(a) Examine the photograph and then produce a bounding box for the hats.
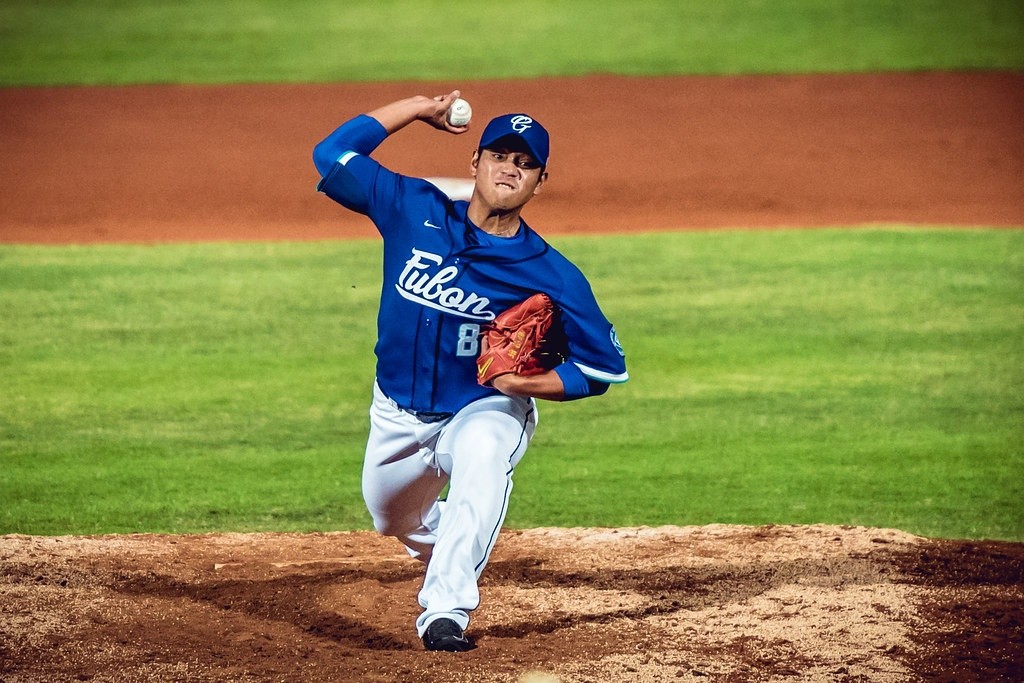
[479,113,550,168]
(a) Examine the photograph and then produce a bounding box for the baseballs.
[445,98,473,127]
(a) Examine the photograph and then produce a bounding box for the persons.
[315,90,629,653]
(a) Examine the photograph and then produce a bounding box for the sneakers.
[423,618,472,651]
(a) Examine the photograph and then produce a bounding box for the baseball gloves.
[473,290,556,390]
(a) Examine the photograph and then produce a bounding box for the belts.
[398,408,452,424]
